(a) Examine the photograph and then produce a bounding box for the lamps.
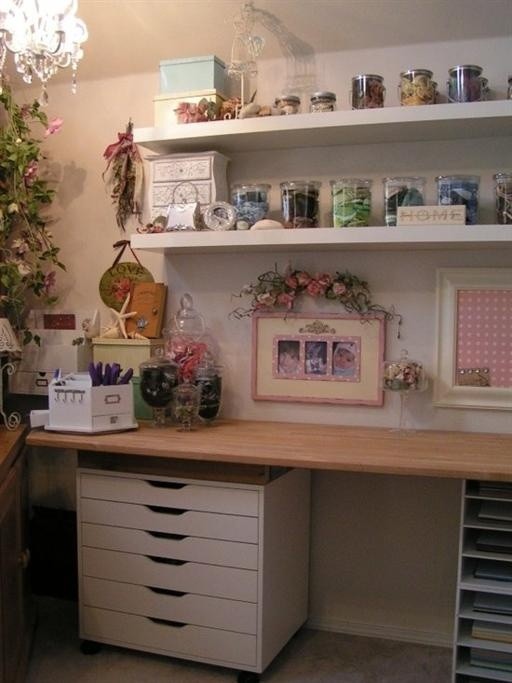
[1,315,24,430]
[1,0,91,105]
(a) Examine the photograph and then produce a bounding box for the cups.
[229,172,512,232]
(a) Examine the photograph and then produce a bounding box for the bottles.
[159,292,219,425]
[274,64,491,113]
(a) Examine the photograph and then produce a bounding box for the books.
[465,478,512,673]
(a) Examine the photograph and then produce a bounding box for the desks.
[26,410,512,682]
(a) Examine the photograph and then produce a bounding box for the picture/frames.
[250,310,389,407]
[430,266,512,409]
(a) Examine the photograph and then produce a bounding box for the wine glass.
[139,347,178,430]
[188,350,223,427]
[174,377,199,433]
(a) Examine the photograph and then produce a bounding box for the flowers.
[172,96,221,123]
[227,256,405,325]
[379,356,429,393]
[0,90,67,365]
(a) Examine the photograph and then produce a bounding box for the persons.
[333,341,358,378]
[305,341,327,374]
[278,340,298,373]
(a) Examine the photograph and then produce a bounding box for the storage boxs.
[151,87,229,124]
[158,54,231,96]
[131,377,155,420]
[90,336,166,375]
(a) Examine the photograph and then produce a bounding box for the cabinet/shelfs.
[145,148,230,229]
[0,426,33,682]
[450,478,512,683]
[130,96,512,251]
[75,465,315,681]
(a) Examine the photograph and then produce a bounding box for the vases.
[396,390,417,437]
